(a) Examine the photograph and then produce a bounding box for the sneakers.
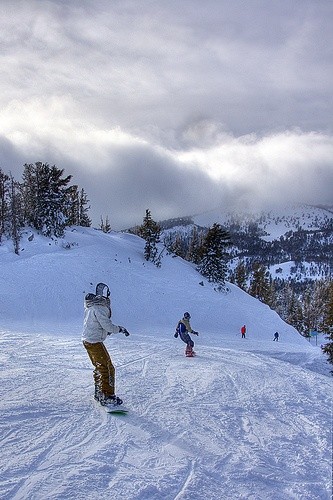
[186,346,193,357]
[95,385,103,402]
[100,395,123,407]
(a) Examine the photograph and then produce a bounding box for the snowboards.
[92,395,129,413]
[185,352,195,357]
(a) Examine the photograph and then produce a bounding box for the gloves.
[118,326,129,336]
[174,333,178,338]
[193,331,198,336]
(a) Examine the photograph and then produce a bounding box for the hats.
[184,312,190,317]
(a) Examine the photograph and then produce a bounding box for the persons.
[240,324,247,338]
[272,331,279,342]
[174,311,198,357]
[80,282,130,408]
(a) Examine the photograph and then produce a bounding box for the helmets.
[96,283,110,298]
